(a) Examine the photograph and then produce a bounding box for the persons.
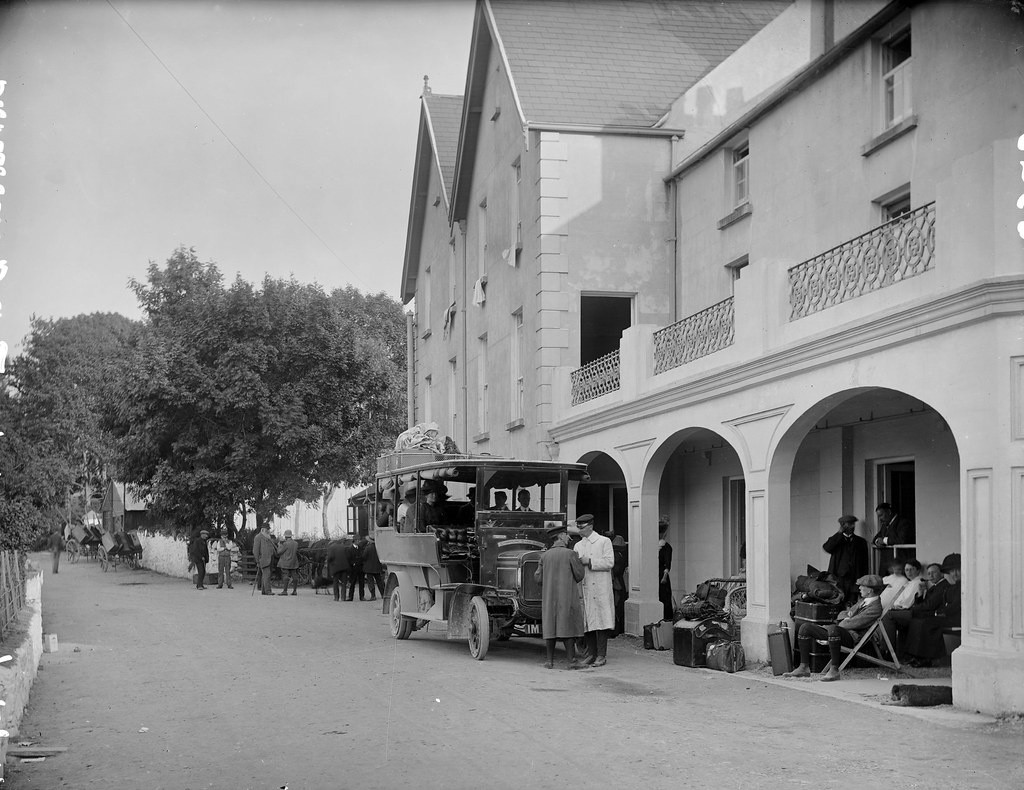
[48,527,63,573]
[659,521,674,620]
[277,530,299,596]
[253,524,276,595]
[192,530,209,590]
[872,503,911,589]
[514,490,533,511]
[534,525,589,669]
[573,514,614,666]
[783,553,961,682]
[602,530,628,640]
[327,535,385,601]
[216,530,233,589]
[740,541,746,566]
[486,491,510,511]
[377,486,449,532]
[822,515,868,604]
[459,487,476,527]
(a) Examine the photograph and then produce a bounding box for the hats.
[421,481,444,494]
[366,533,375,543]
[856,575,883,587]
[260,524,293,536]
[547,514,593,539]
[405,490,416,497]
[466,488,475,497]
[839,515,857,523]
[940,554,961,571]
[200,530,227,534]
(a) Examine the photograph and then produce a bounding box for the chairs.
[815,583,916,678]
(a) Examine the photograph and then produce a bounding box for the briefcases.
[643,619,675,651]
[767,621,793,676]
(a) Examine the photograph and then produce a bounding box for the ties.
[846,537,850,543]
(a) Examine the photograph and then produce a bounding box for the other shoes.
[580,655,596,667]
[198,585,297,596]
[905,657,937,668]
[821,670,840,681]
[564,658,589,670]
[783,664,810,677]
[334,597,383,601]
[592,656,607,667]
[544,662,552,668]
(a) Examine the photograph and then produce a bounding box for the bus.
[369,459,581,662]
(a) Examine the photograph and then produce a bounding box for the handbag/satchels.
[696,580,727,608]
[706,639,745,672]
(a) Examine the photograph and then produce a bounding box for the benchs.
[426,524,479,562]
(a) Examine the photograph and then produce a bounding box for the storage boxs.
[671,617,732,669]
[378,449,433,473]
